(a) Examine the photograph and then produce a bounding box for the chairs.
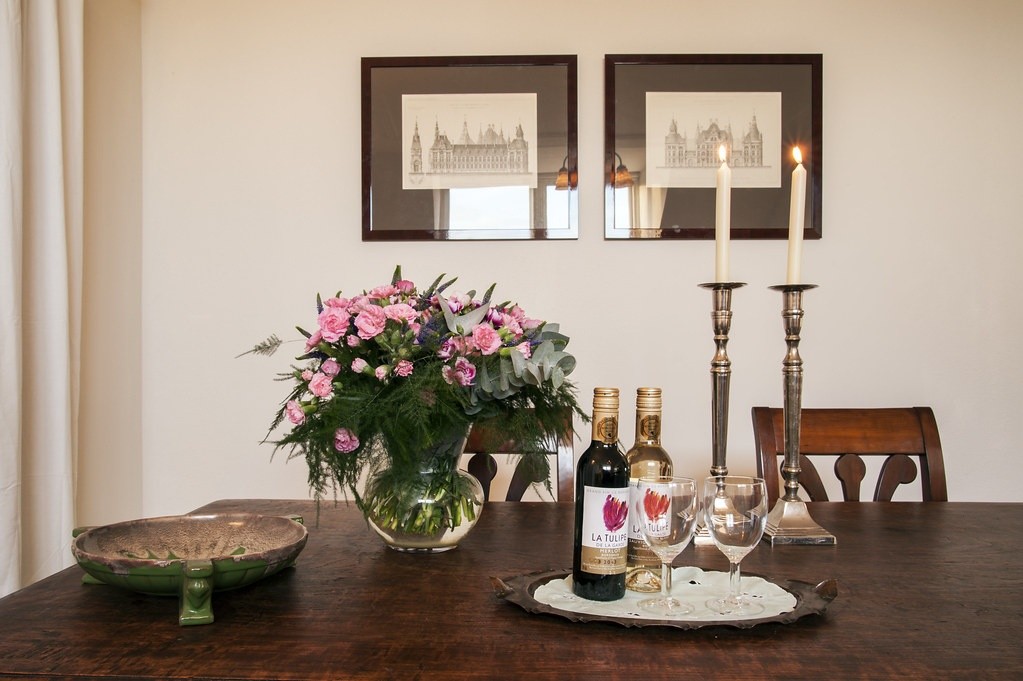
[752,407,948,502]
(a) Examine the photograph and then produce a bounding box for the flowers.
[235,265,593,535]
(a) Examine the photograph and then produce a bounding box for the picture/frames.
[605,54,822,240]
[361,55,578,242]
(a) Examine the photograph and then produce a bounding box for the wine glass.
[636,477,700,617]
[704,476,768,615]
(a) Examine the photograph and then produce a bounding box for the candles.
[786,145,807,284]
[716,145,731,282]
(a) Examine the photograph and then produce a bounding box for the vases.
[362,423,485,552]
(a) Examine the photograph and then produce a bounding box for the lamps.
[611,151,635,187]
[555,156,577,190]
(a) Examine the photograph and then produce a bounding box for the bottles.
[622,387,673,592]
[571,388,630,602]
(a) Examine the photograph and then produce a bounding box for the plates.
[71,513,310,626]
[489,567,838,630]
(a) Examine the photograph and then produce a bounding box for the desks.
[0,499,1023,681]
